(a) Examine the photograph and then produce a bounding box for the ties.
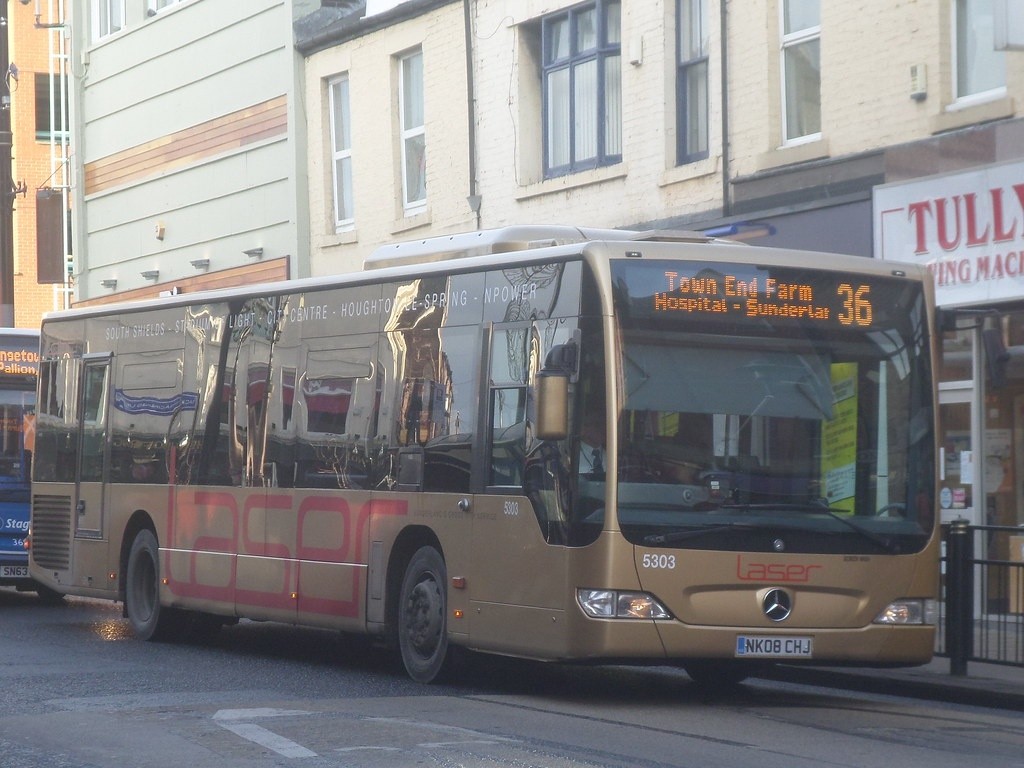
[592,449,604,474]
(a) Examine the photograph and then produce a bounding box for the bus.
[0,327,69,599]
[29,236,1012,695]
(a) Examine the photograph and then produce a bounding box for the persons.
[578,409,607,474]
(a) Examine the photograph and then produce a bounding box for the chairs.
[752,467,806,502]
[715,456,759,475]
[3,449,32,476]
